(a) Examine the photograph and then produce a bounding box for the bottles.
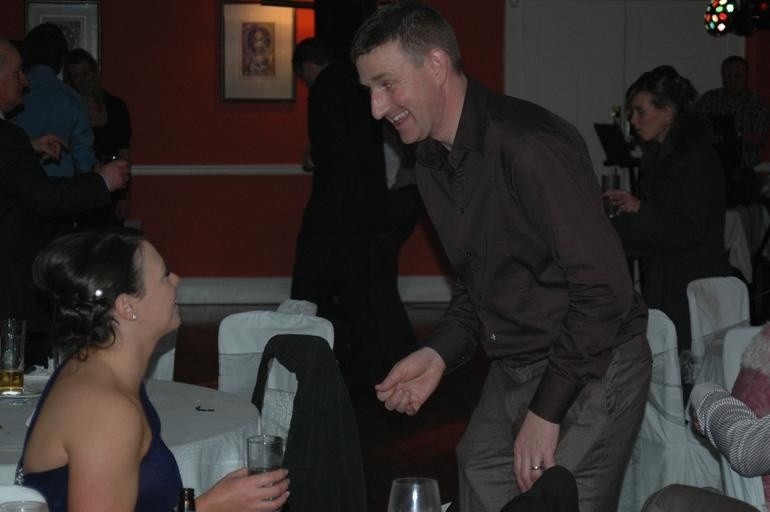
[176,488,196,512]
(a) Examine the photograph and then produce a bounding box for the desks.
[0,374,264,511]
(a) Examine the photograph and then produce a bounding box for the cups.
[602,174,621,220]
[388,478,441,512]
[0,318,27,395]
[0,500,48,512]
[246,435,284,512]
[106,150,128,164]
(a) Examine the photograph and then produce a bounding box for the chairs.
[250,333,370,512]
[718,326,766,508]
[217,311,337,402]
[617,306,724,511]
[140,302,179,379]
[686,274,751,386]
[0,485,49,511]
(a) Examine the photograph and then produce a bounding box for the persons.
[293,38,424,368]
[603,57,769,512]
[13,227,292,512]
[2,23,131,374]
[346,0,653,510]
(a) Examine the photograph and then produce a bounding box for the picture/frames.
[24,0,102,78]
[218,1,298,104]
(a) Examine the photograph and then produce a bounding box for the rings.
[531,465,541,469]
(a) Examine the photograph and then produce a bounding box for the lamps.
[703,0,742,38]
